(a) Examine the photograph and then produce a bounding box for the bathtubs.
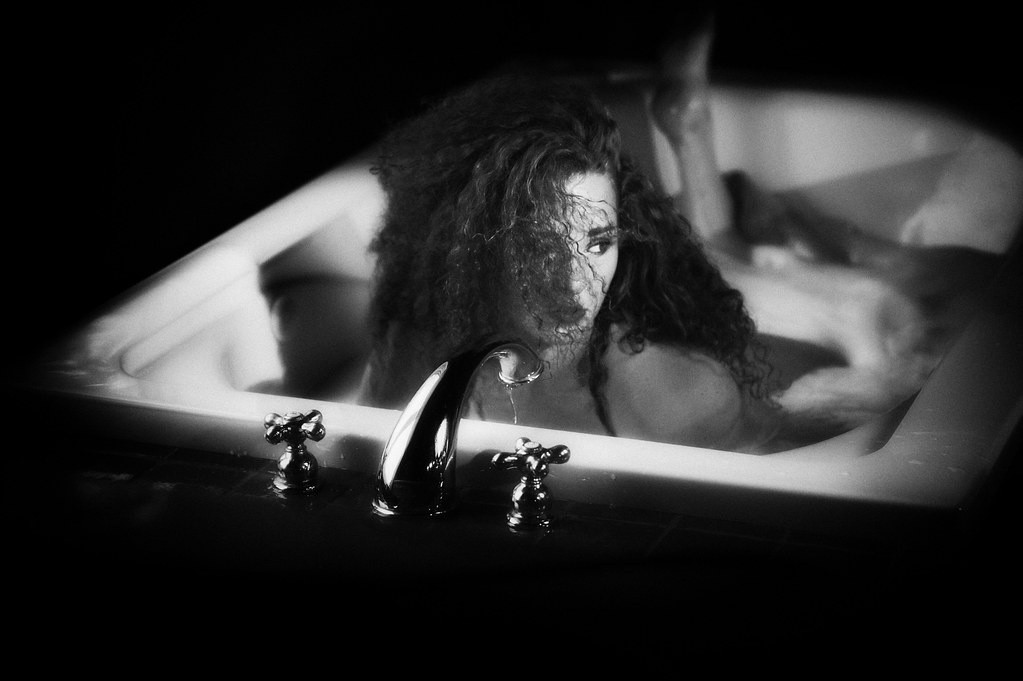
[28,76,1021,518]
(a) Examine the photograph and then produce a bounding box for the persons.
[362,19,769,447]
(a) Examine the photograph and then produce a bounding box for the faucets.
[366,330,547,523]
